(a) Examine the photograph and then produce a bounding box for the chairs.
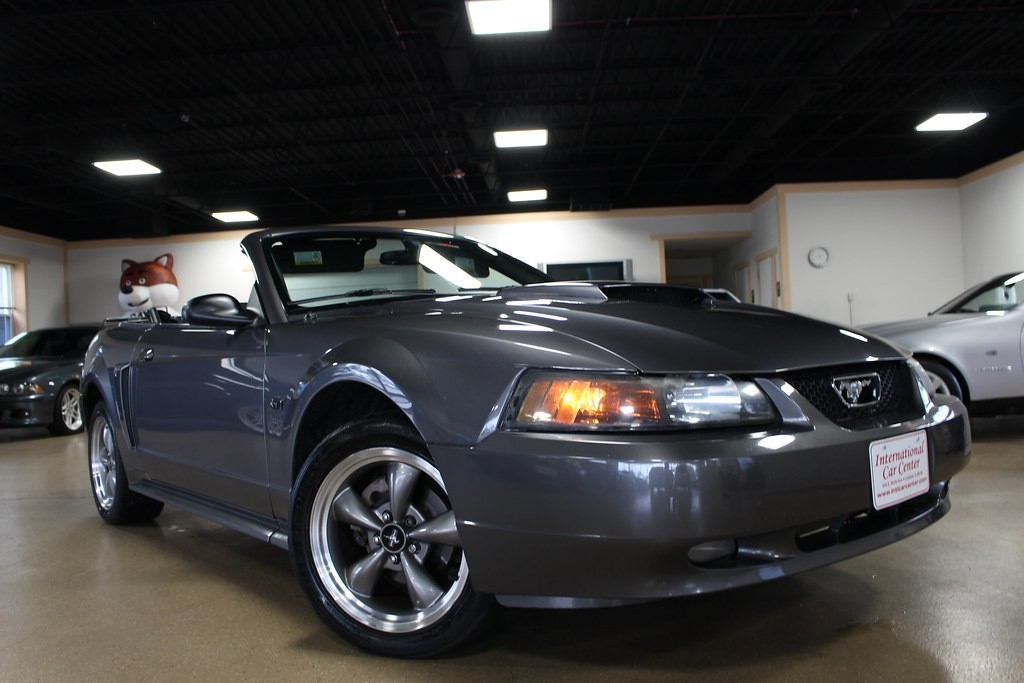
[181,293,245,327]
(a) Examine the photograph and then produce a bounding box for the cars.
[852,268,1024,417]
[0,318,114,434]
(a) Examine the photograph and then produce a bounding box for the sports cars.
[77,219,972,661]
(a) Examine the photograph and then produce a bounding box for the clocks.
[808,246,831,268]
